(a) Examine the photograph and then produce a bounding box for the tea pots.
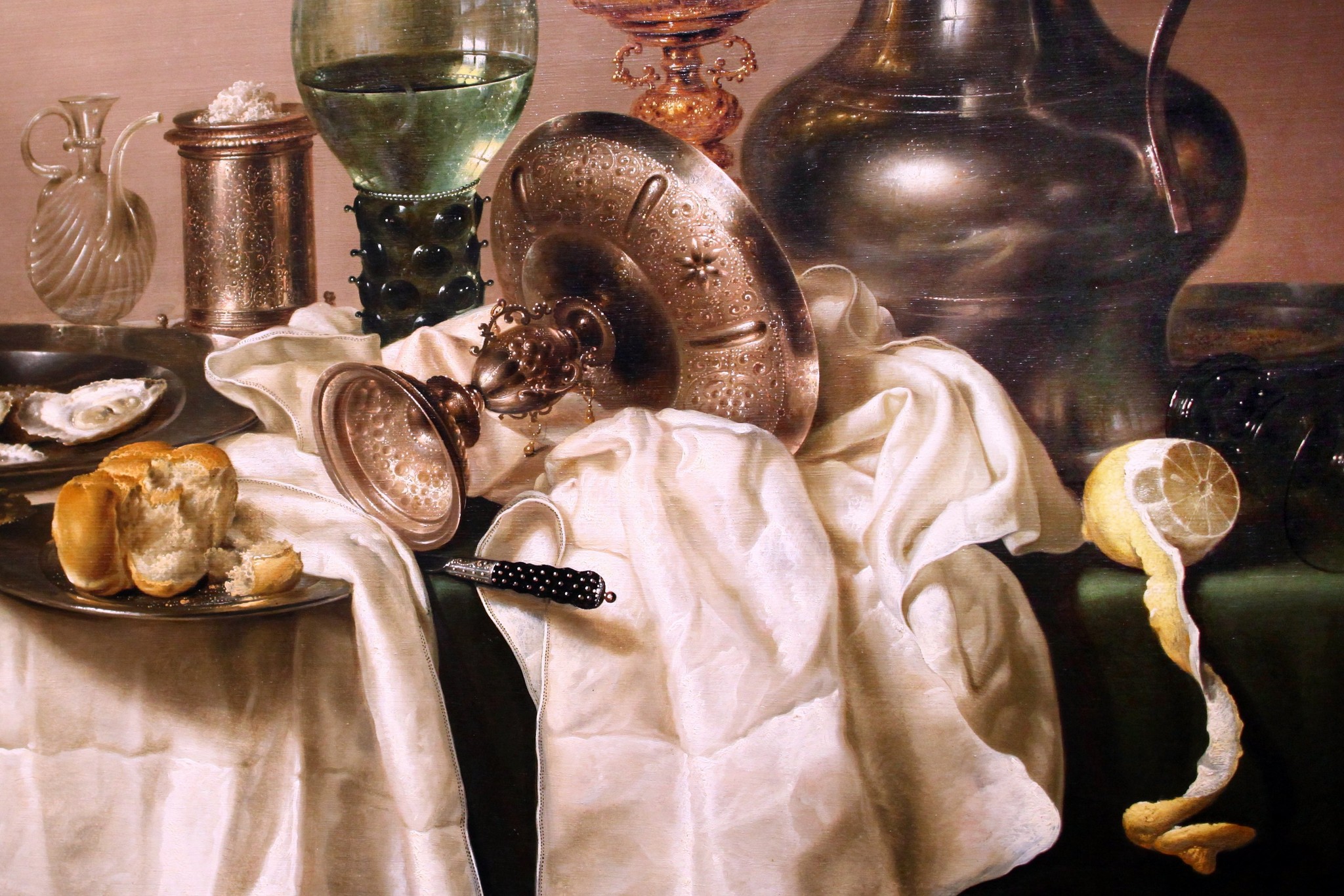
[20,93,161,327]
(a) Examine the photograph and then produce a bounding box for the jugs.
[737,0,1247,496]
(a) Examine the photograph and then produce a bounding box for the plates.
[1168,279,1344,365]
[0,502,353,622]
[0,321,257,476]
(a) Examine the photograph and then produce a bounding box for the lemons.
[1081,437,1239,569]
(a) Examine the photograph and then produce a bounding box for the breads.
[53,440,305,598]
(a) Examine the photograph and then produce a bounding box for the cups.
[165,102,323,337]
